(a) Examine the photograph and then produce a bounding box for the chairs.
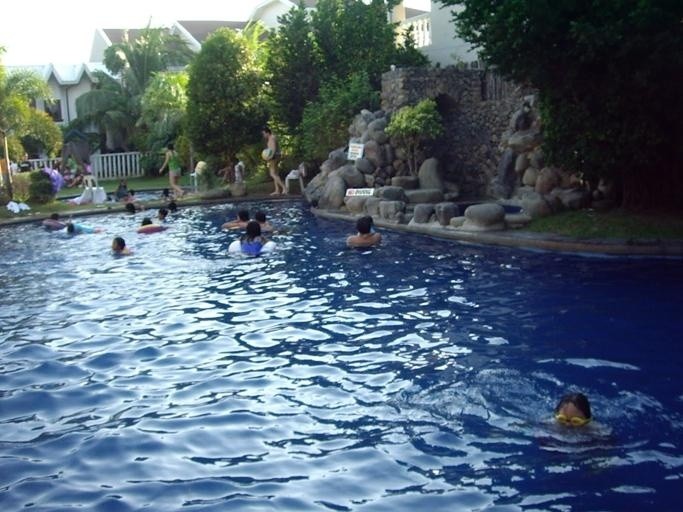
[82,176,105,205]
[284,162,307,194]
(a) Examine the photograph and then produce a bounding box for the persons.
[238,210,274,232]
[346,216,382,249]
[510,100,531,134]
[261,127,286,197]
[48,213,101,234]
[552,392,594,428]
[115,179,179,227]
[239,221,268,256]
[18,152,32,172]
[217,154,247,185]
[223,209,254,230]
[52,154,92,189]
[109,237,130,256]
[158,145,187,199]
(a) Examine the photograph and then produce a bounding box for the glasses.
[555,414,590,426]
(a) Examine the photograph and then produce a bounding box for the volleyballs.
[262,149,273,161]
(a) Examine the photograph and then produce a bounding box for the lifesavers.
[136,224,166,233]
[42,218,64,230]
[227,240,276,256]
